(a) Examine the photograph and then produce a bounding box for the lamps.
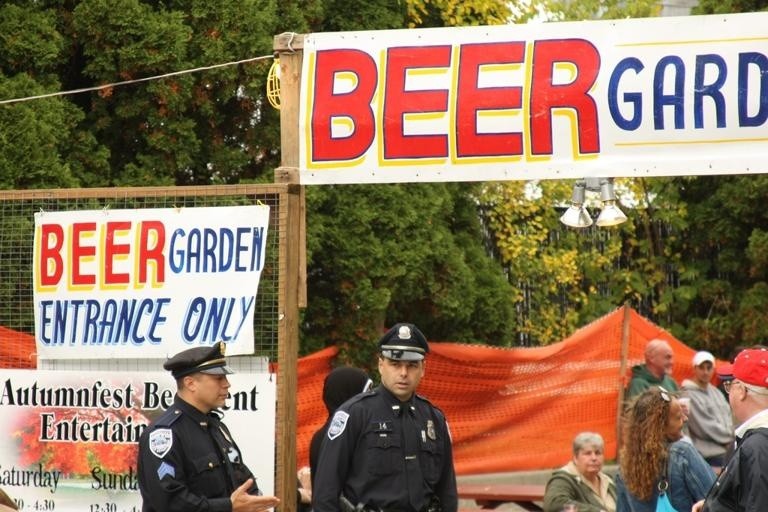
[558,177,627,229]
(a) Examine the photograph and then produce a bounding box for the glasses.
[658,385,671,412]
[723,380,743,394]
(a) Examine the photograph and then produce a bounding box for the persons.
[543,338,768,512]
[309,366,374,485]
[296,466,315,505]
[136,342,282,512]
[310,322,459,512]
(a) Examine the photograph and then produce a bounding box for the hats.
[716,347,768,389]
[379,322,429,361]
[693,350,715,367]
[163,341,235,379]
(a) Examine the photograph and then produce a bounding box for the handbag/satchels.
[654,487,678,512]
